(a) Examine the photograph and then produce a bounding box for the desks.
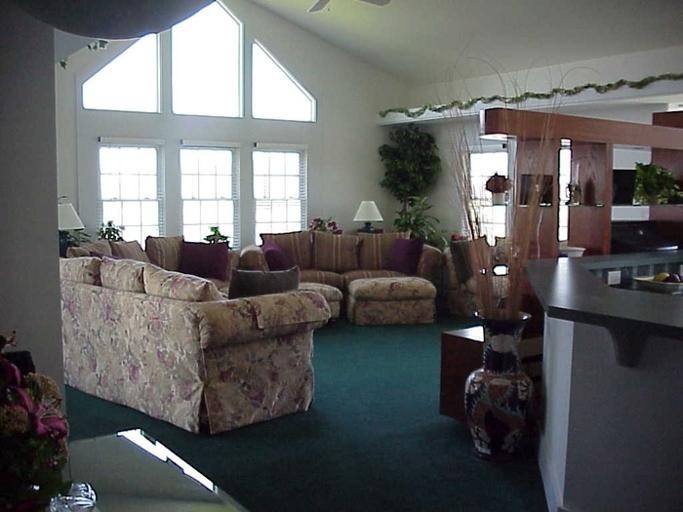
[357,229,383,233]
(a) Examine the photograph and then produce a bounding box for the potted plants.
[484,171,512,206]
[377,121,442,238]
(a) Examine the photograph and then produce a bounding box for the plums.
[665,272,681,282]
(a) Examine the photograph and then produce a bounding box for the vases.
[0,438,66,511]
[464,310,533,463]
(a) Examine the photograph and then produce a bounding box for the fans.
[307,0,392,13]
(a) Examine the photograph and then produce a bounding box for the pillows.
[263,241,293,270]
[390,240,418,274]
[229,265,300,307]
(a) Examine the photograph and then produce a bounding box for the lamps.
[351,198,385,231]
[58,203,85,257]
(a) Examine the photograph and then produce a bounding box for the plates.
[633,274,682,291]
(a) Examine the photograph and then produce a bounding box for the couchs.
[445,235,511,319]
[66,236,240,294]
[60,257,330,436]
[240,231,442,319]
[347,278,438,325]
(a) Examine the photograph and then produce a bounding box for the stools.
[298,280,343,323]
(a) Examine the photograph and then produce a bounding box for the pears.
[655,272,670,281]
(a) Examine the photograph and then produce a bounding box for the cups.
[490,191,511,206]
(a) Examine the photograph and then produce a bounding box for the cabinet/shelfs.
[439,324,540,440]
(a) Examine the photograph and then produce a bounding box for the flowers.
[0,331,73,462]
[311,216,343,236]
